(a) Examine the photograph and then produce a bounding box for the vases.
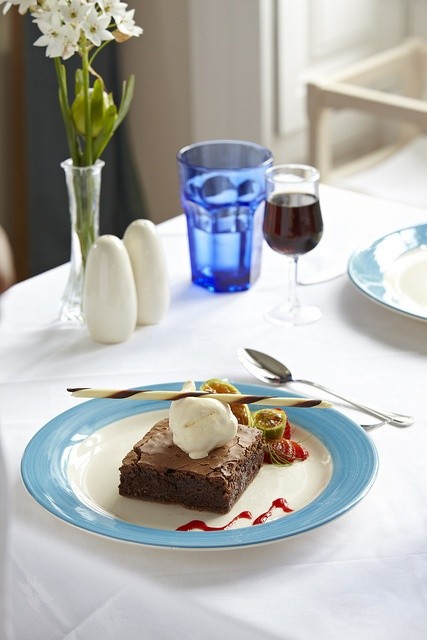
[56,156,106,325]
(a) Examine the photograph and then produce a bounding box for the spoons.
[238,346,416,427]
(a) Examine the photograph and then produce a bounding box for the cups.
[174,138,273,294]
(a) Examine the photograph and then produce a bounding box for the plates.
[345,219,427,322]
[19,376,381,552]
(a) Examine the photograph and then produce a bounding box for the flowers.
[0,0,143,165]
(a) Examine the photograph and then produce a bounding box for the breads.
[67,387,334,514]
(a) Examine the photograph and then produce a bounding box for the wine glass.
[262,163,324,329]
